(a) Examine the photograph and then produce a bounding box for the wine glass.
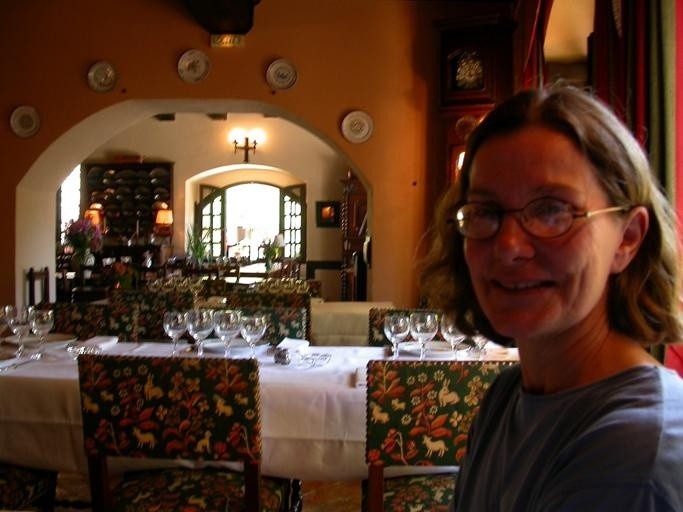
[160,307,267,358]
[382,312,488,362]
[3,304,53,361]
[144,276,203,300]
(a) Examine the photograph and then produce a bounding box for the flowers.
[64,218,102,254]
[260,233,284,276]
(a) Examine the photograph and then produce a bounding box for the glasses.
[450,195,626,240]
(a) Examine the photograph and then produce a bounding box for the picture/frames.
[314,200,341,229]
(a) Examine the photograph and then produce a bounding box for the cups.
[253,276,310,294]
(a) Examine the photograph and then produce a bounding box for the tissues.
[274,336,312,366]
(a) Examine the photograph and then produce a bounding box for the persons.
[409,82,682,511]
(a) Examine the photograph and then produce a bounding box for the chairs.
[365,305,521,511]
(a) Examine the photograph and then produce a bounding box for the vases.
[68,245,95,279]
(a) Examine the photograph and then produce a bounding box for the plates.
[198,335,270,354]
[396,339,470,357]
[5,333,77,351]
[88,165,170,233]
